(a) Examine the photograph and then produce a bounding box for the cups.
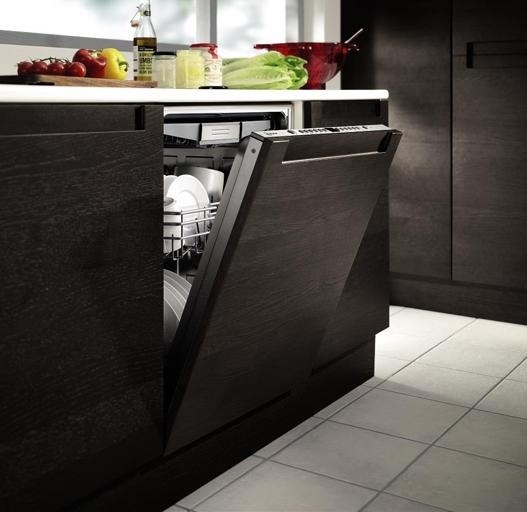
[151,50,177,88]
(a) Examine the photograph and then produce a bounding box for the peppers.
[101,47,129,80]
[72,48,107,78]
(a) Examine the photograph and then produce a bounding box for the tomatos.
[17,60,87,77]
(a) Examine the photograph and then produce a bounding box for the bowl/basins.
[254,42,359,90]
[174,166,225,202]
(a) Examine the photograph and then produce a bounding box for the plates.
[157,161,211,348]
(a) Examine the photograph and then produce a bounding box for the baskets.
[163,202,221,260]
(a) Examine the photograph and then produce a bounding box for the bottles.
[131,0,158,83]
[188,42,222,88]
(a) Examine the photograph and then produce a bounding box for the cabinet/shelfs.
[1,102,391,511]
[341,0,525,327]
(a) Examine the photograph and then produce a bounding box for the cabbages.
[222,51,308,90]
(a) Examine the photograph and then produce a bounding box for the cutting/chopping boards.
[0,73,159,88]
